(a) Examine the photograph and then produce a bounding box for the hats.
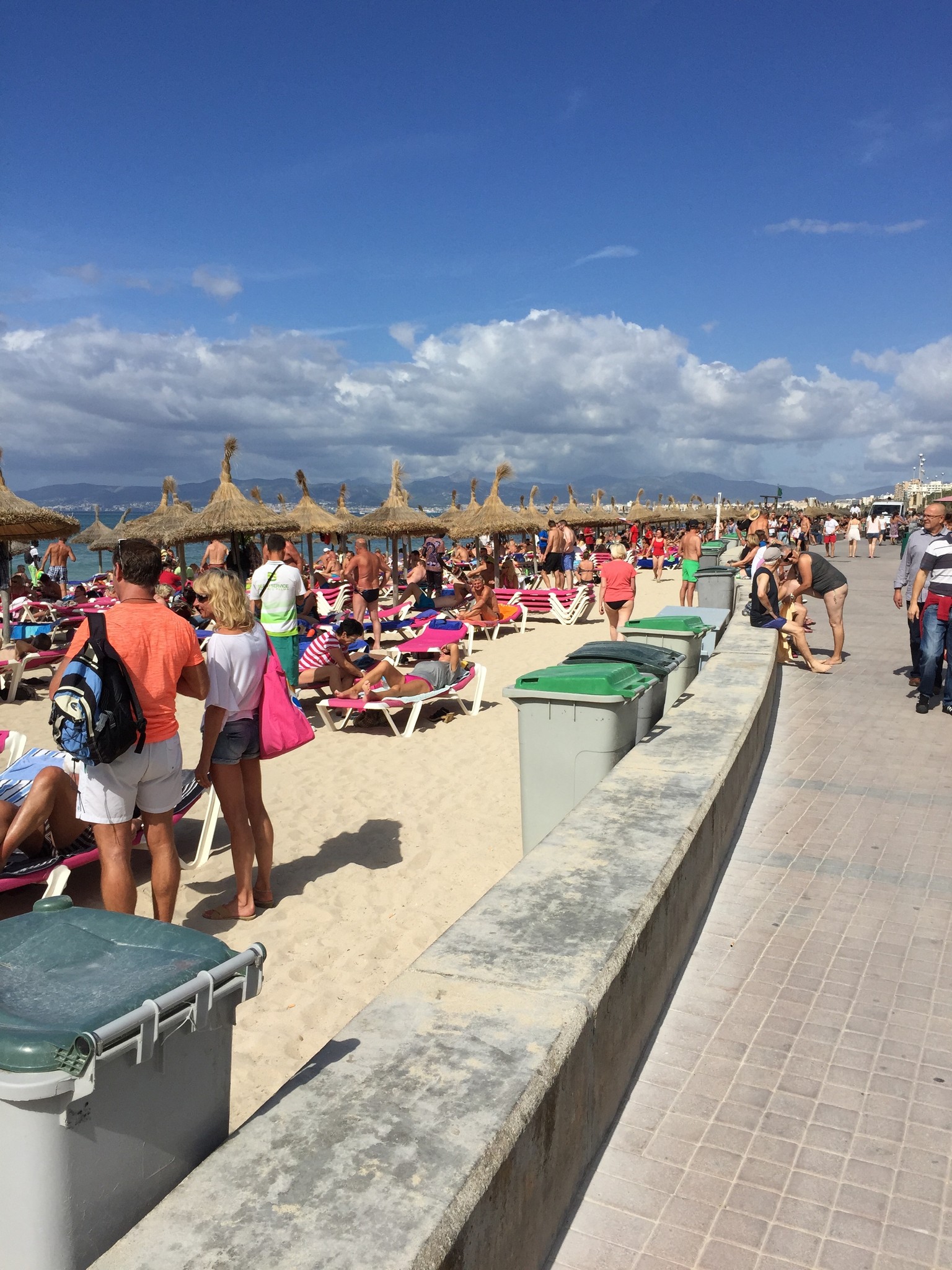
[763,546,786,562]
[748,508,761,521]
[323,548,331,552]
[689,520,698,527]
[185,581,193,588]
[487,557,495,565]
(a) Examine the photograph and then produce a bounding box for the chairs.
[0,729,27,770]
[315,661,488,737]
[0,769,220,904]
[0,515,674,704]
[1,748,83,808]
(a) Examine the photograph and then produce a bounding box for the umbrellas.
[933,496,952,502]
[2,436,847,609]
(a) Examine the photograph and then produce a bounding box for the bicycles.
[897,523,907,543]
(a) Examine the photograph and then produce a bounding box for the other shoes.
[788,640,800,658]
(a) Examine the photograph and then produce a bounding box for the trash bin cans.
[720,534,740,550]
[694,566,742,626]
[700,541,726,556]
[732,531,747,547]
[698,548,722,569]
[557,642,687,745]
[502,663,660,859]
[1,895,265,1270]
[709,540,730,546]
[616,616,716,717]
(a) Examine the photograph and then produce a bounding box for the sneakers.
[942,704,952,715]
[909,677,921,686]
[916,694,930,714]
[932,687,941,695]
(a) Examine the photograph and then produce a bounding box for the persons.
[192,569,276,920]
[893,503,952,695]
[1,752,143,870]
[905,514,910,525]
[943,513,952,531]
[910,511,920,527]
[908,531,952,716]
[857,504,861,521]
[904,512,909,522]
[1,510,887,706]
[850,503,860,520]
[889,511,909,545]
[894,510,904,528]
[917,512,922,521]
[47,537,210,922]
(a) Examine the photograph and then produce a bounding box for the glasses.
[11,580,18,583]
[501,567,507,569]
[348,636,357,643]
[486,561,493,564]
[784,549,793,563]
[922,514,941,519]
[196,592,209,601]
[480,551,485,553]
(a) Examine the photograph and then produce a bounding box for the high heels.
[435,707,455,722]
[427,710,450,723]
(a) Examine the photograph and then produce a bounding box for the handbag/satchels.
[248,541,263,570]
[258,655,314,760]
[226,547,250,580]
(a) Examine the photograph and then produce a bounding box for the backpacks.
[24,547,36,564]
[48,613,146,766]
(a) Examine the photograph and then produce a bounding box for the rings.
[197,779,201,782]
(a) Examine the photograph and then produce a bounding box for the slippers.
[202,906,257,921]
[803,618,816,633]
[234,894,274,909]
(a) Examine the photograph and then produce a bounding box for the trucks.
[864,497,909,540]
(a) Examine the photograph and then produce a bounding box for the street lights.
[934,475,938,499]
[925,477,930,500]
[940,472,945,499]
[911,467,916,498]
[917,453,926,496]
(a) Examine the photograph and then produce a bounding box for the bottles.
[382,674,390,690]
[526,569,529,576]
